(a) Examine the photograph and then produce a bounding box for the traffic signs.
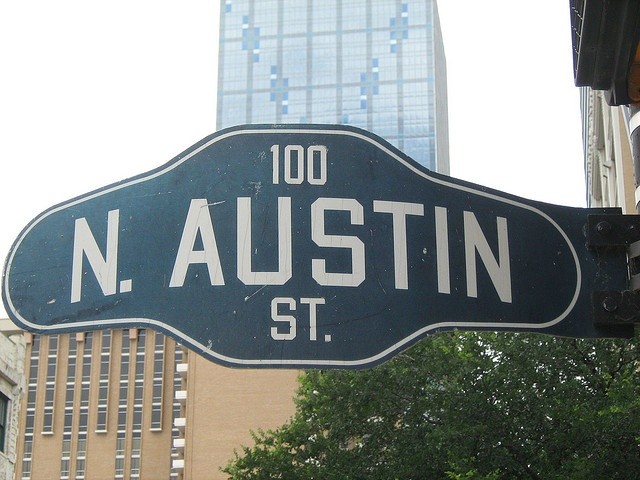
[2,122,639,369]
